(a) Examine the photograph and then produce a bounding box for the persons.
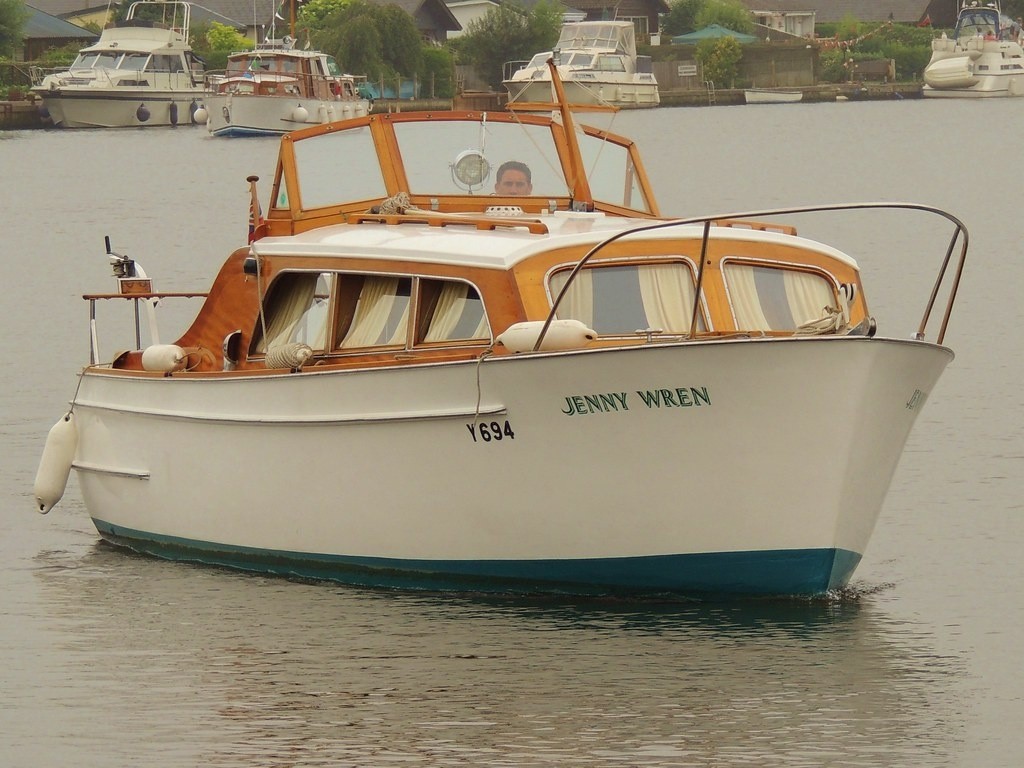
[495,161,533,196]
[974,17,1022,42]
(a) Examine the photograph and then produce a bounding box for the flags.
[917,16,930,29]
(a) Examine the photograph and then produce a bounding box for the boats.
[202,0,374,136]
[743,87,804,102]
[36,56,971,593]
[32,0,223,128]
[921,0,1024,99]
[506,23,661,108]
